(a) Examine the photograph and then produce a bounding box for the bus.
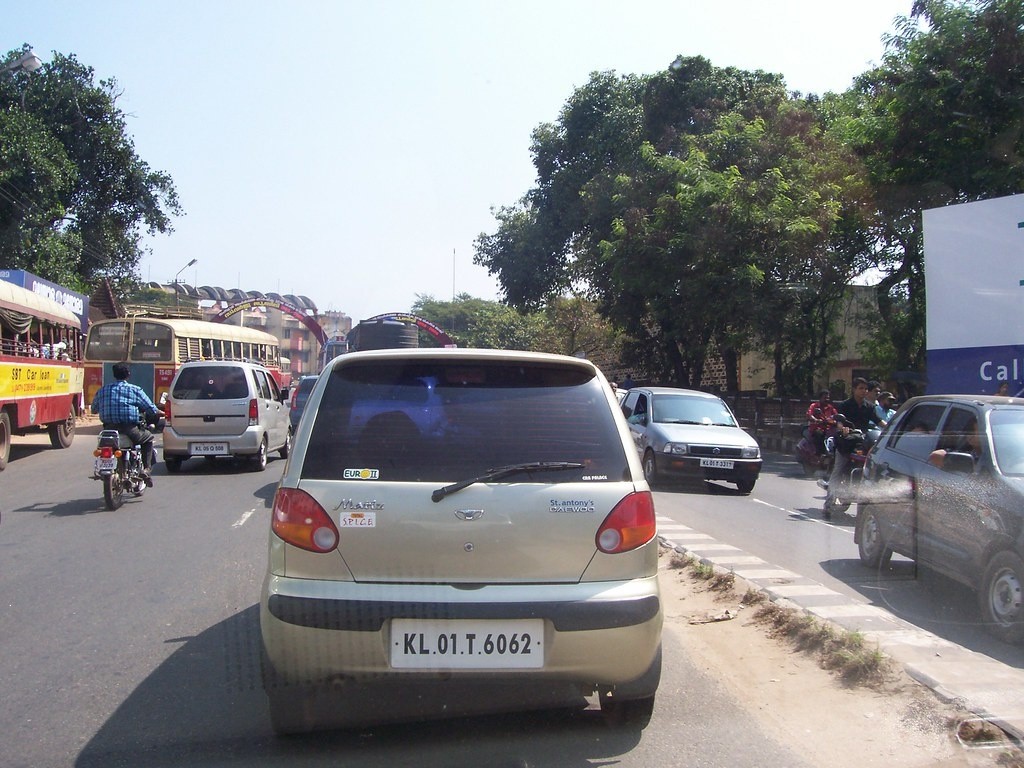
[0,280,83,470]
[318,336,356,376]
[83,317,283,428]
[275,357,293,390]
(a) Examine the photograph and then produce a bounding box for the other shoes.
[824,505,833,516]
[141,468,153,487]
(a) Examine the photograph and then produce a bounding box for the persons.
[995,382,1010,397]
[907,422,929,433]
[610,382,620,403]
[930,417,982,466]
[805,378,899,516]
[90,362,159,488]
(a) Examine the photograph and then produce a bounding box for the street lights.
[175,258,197,319]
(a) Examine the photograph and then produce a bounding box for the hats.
[113,362,132,378]
[877,391,896,402]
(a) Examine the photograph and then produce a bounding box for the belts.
[103,423,133,427]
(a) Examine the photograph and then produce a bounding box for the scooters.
[794,407,839,477]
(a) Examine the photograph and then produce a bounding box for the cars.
[619,386,764,493]
[163,360,293,474]
[614,389,645,417]
[259,350,664,734]
[289,375,318,433]
[347,377,450,463]
[853,394,1024,646]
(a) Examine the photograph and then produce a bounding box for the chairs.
[358,411,425,469]
[223,383,246,397]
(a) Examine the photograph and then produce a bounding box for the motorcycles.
[88,392,169,510]
[816,415,882,509]
